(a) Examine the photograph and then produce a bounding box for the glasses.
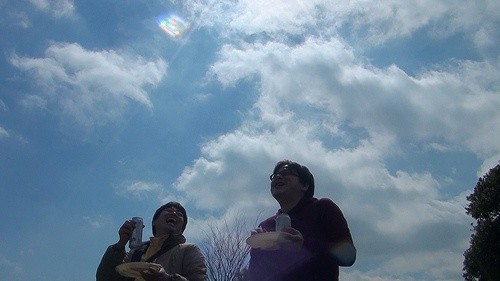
[270,170,300,181]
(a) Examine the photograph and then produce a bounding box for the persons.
[245,159,357,281]
[96,201,210,281]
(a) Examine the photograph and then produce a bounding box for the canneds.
[276,212,291,235]
[129,216,143,248]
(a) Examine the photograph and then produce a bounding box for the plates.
[116,262,162,279]
[246,232,292,251]
